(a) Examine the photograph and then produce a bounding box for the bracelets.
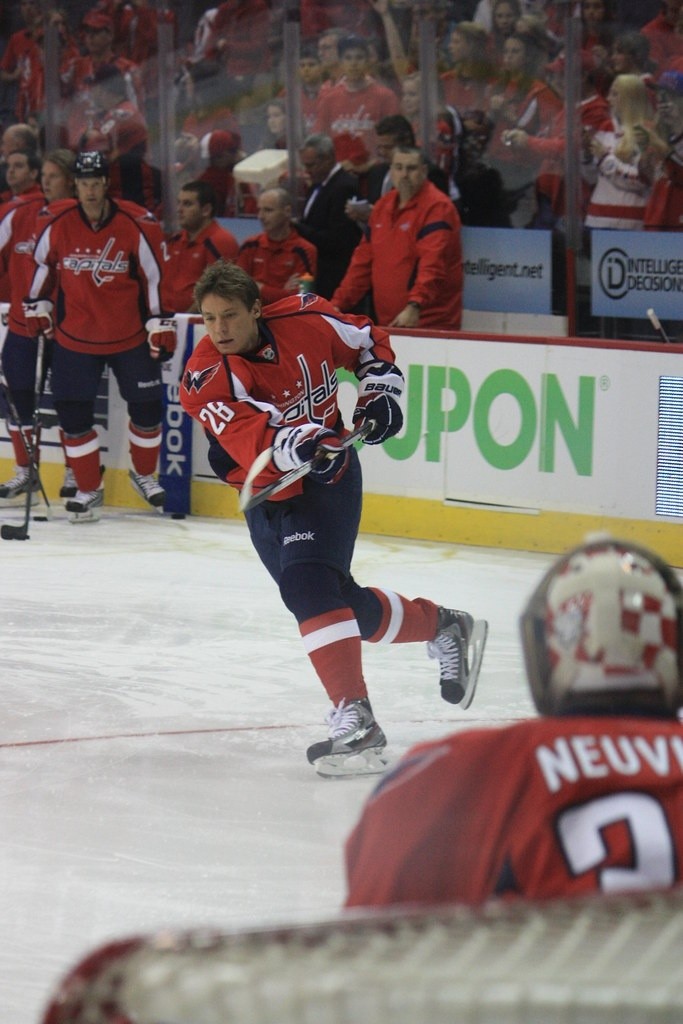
[408,302,419,307]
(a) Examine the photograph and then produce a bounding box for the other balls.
[172,514,185,519]
[33,516,47,521]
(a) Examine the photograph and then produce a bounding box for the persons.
[344,541,683,905]
[0,148,178,523]
[0,0,683,336]
[330,145,463,332]
[178,258,489,778]
[236,189,317,305]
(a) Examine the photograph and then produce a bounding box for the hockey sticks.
[0,357,67,518]
[0,333,46,540]
[236,417,377,514]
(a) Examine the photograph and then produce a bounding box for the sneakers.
[427,607,490,713]
[62,478,106,523]
[58,466,80,508]
[0,463,42,508]
[127,467,166,513]
[304,697,391,778]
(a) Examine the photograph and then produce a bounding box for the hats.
[199,129,234,160]
[81,13,117,37]
[643,71,683,95]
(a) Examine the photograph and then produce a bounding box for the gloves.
[273,423,349,486]
[143,315,180,363]
[352,362,404,446]
[21,294,57,342]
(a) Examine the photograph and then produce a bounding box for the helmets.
[518,534,683,718]
[72,149,111,178]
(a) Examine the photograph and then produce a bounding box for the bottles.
[580,125,593,165]
[297,272,314,295]
